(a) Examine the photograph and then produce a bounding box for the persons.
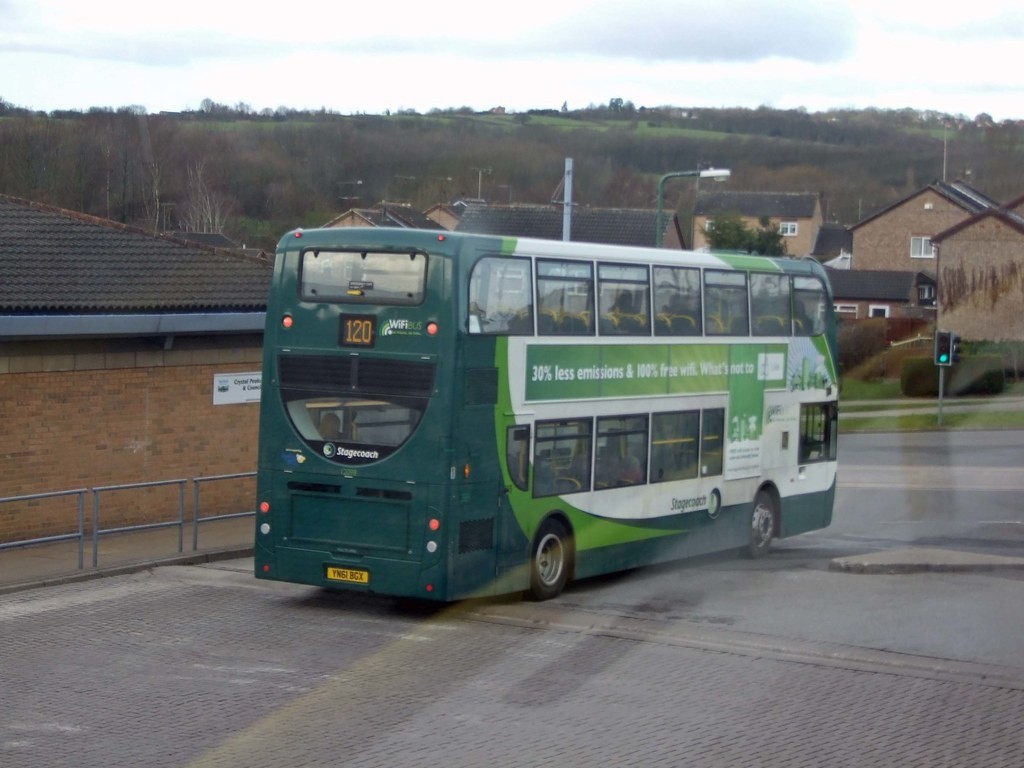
[794,300,813,334]
[611,443,641,479]
[678,294,702,330]
[320,413,341,439]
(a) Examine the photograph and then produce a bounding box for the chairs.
[525,451,640,497]
[471,303,804,336]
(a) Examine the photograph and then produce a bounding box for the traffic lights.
[950,335,961,362]
[935,330,952,366]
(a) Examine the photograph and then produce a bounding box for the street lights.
[656,167,731,249]
[439,177,452,216]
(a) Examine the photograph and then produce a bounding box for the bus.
[253,227,840,601]
